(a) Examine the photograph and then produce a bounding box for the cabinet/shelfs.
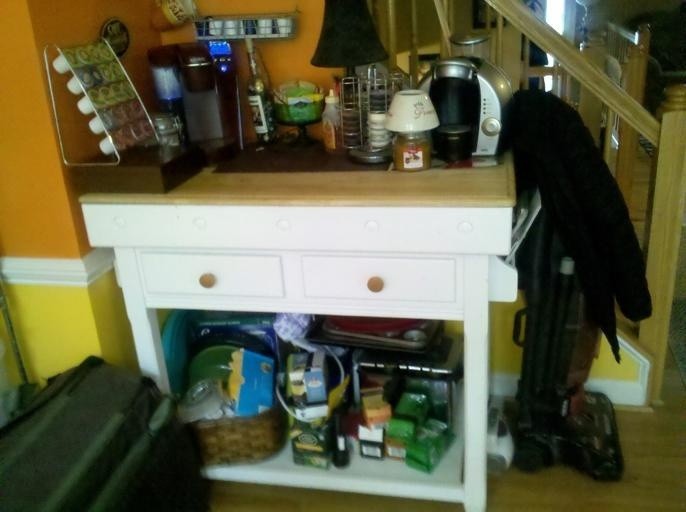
[77,150,519,511]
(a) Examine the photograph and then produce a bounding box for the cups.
[340,91,391,149]
[384,90,440,133]
[150,0,296,37]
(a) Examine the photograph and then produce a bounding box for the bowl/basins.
[273,100,323,124]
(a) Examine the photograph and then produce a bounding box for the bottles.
[429,62,479,155]
[394,125,474,172]
[331,409,349,467]
[244,38,277,146]
[147,46,212,103]
[322,88,342,151]
